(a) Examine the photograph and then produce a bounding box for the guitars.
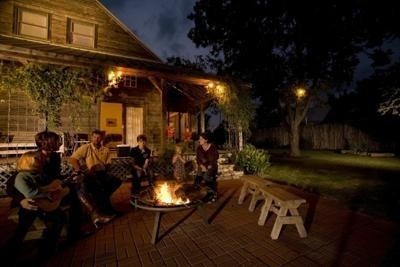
[35,171,78,212]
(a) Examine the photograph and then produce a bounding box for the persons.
[0,130,122,267]
[129,135,151,193]
[194,132,219,204]
[171,145,186,179]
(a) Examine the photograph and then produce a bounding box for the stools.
[238,175,272,212]
[258,187,308,240]
[127,173,161,189]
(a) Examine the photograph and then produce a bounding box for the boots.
[79,194,114,228]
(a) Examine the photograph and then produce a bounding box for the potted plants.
[233,143,272,178]
